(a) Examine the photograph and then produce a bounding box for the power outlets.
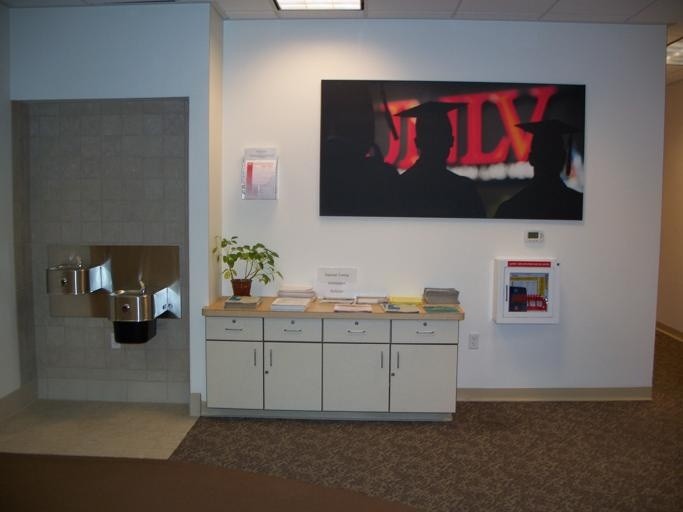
[468,334,479,350]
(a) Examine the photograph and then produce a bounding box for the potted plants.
[212,236,284,296]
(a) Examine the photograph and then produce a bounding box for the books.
[273,297,311,312]
[224,295,264,309]
[334,305,373,313]
[425,286,459,303]
[276,284,316,299]
[356,294,389,304]
[322,296,354,304]
[390,296,422,304]
[381,302,420,318]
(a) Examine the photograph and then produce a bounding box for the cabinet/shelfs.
[202,317,322,422]
[322,319,459,422]
